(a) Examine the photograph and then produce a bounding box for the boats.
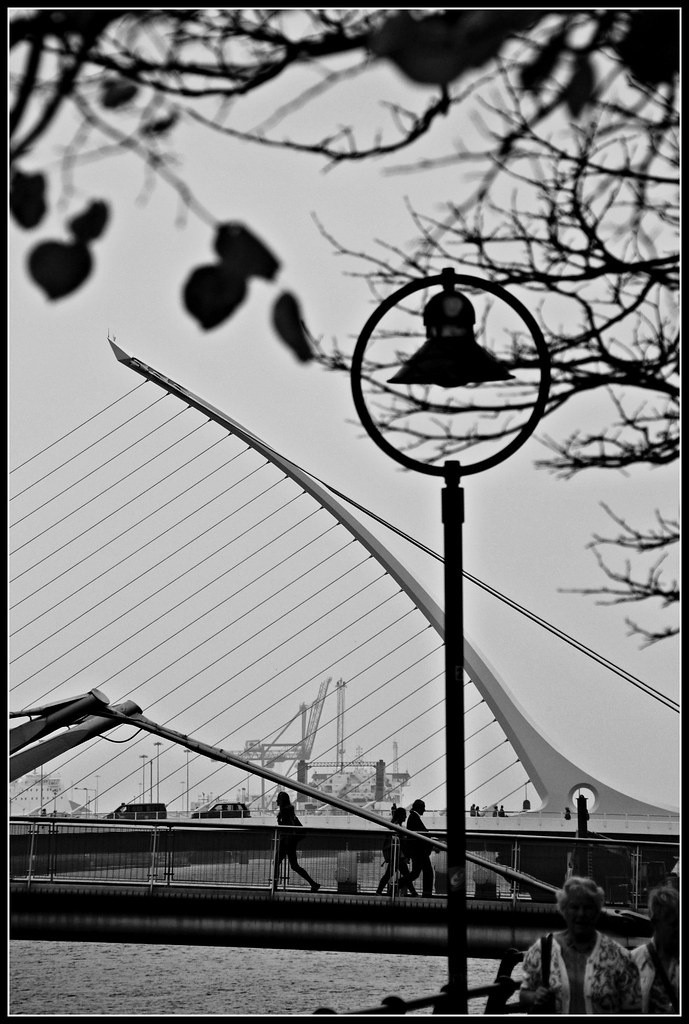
[274,769,412,816]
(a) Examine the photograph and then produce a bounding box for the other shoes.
[311,884,320,893]
[376,891,383,896]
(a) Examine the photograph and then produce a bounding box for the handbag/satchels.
[295,816,306,843]
[527,932,555,1014]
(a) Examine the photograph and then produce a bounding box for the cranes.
[392,740,398,772]
[335,678,347,775]
[210,677,332,763]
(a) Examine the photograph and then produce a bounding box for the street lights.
[153,741,162,803]
[184,749,191,817]
[38,738,46,813]
[74,787,96,819]
[139,754,148,803]
[180,781,186,810]
[94,775,102,813]
[349,266,550,1015]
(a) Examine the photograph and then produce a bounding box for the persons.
[470,804,480,817]
[273,792,320,892]
[519,876,679,1015]
[376,799,440,896]
[493,805,505,817]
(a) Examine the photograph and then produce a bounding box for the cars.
[191,802,251,818]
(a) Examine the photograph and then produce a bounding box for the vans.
[103,803,167,819]
